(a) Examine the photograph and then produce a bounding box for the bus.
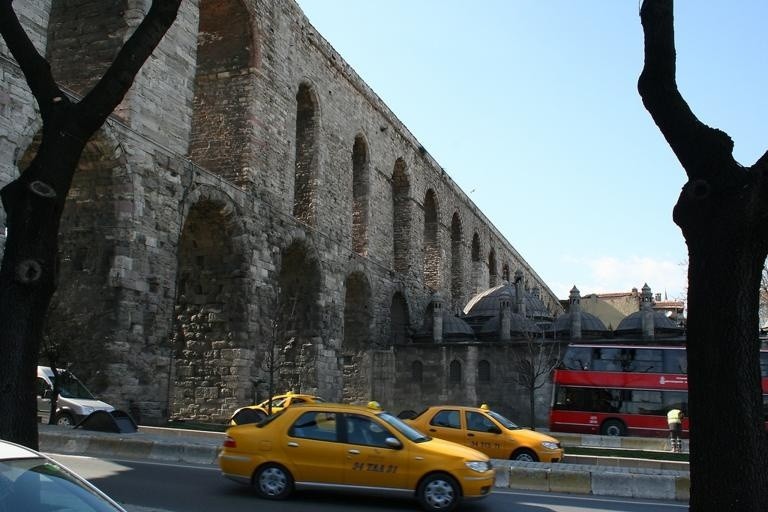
[546,341,768,439]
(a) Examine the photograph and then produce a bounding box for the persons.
[667,409,684,453]
[360,419,384,444]
[469,414,489,432]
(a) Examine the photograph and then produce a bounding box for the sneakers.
[671,447,681,452]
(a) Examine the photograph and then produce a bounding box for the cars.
[396,403,567,465]
[0,438,130,512]
[225,390,334,430]
[216,399,498,509]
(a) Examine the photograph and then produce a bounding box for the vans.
[35,364,117,431]
